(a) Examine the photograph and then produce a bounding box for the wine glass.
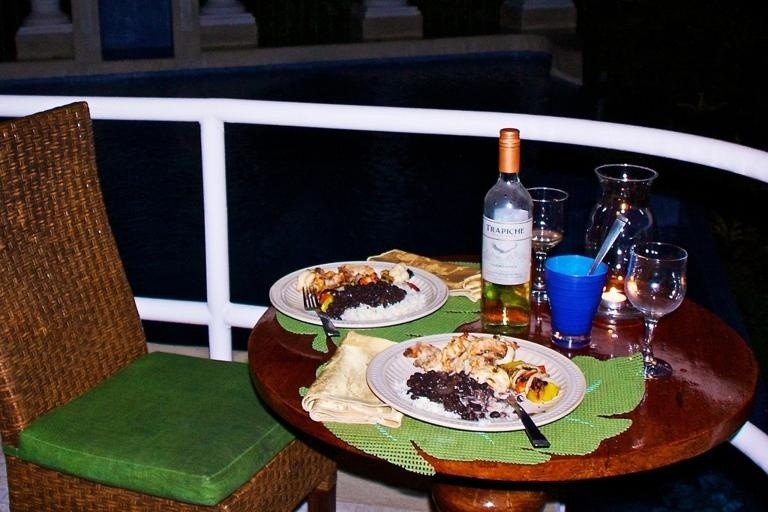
[520,162,690,382]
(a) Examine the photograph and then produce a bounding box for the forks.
[301,284,341,338]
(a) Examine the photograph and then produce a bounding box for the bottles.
[481,127,536,337]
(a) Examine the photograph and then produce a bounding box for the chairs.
[0,99,344,512]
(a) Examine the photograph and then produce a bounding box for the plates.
[268,259,449,330]
[367,332,588,430]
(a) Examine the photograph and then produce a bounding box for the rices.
[395,378,520,425]
[339,282,426,321]
